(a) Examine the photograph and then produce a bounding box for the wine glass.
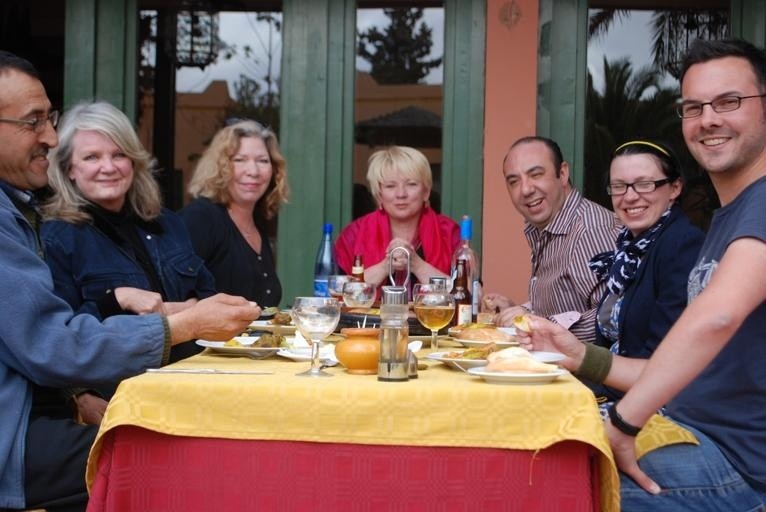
[411,283,451,304]
[415,293,456,361]
[343,282,377,307]
[292,297,341,377]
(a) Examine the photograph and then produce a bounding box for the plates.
[432,348,568,371]
[249,320,296,335]
[453,334,521,351]
[467,364,567,383]
[194,335,287,360]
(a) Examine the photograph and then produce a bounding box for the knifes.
[146,368,273,375]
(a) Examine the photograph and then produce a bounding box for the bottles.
[449,259,473,328]
[454,215,479,323]
[348,255,367,283]
[314,224,338,297]
[378,304,410,381]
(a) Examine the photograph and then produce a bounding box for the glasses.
[605,178,674,196]
[676,95,764,118]
[0,110,59,133]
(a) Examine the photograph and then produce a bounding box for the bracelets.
[608,404,642,436]
[573,341,612,385]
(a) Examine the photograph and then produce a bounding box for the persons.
[335,146,460,301]
[40,102,217,402]
[481,137,625,343]
[176,119,289,321]
[573,139,705,404]
[516,39,766,512]
[0,51,262,512]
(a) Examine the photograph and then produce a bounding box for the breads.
[487,347,535,360]
[485,356,539,374]
[457,325,514,340]
